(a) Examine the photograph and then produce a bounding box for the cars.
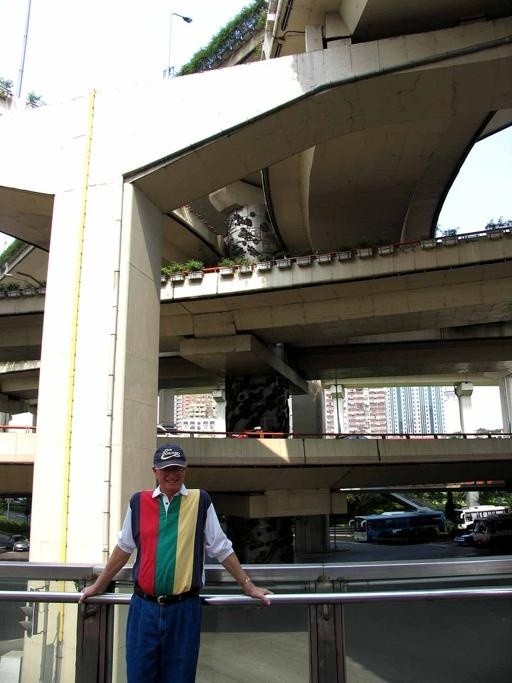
[231,426,278,437]
[340,432,369,439]
[156,421,193,436]
[455,529,473,544]
[7,534,30,552]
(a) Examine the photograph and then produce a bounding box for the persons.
[78,443,274,683]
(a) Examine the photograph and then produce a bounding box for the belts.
[133,587,199,602]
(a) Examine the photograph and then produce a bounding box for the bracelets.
[239,576,252,585]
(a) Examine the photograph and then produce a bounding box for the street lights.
[166,10,192,79]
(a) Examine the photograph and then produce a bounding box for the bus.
[347,508,448,543]
[473,513,512,548]
[453,502,510,532]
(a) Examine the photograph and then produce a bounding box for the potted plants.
[317,238,396,263]
[218,247,313,276]
[420,229,460,249]
[0,280,46,298]
[484,218,505,238]
[160,257,208,282]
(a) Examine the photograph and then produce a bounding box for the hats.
[153,444,188,469]
